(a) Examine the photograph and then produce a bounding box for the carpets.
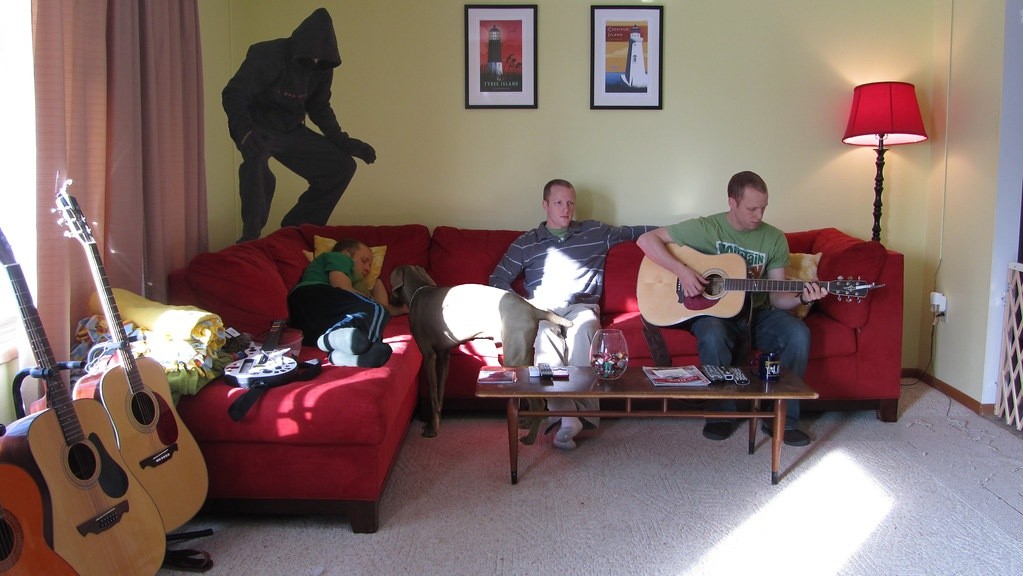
[873,415,1023,545]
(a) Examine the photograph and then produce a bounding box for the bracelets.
[795,292,814,306]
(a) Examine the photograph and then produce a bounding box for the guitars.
[223,320,299,388]
[0,228,166,576]
[51,190,209,534]
[0,423,80,576]
[635,242,869,328]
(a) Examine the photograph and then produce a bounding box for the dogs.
[388,265,573,446]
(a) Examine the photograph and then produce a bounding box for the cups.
[750,352,781,382]
[589,329,629,379]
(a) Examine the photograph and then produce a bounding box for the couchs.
[428,226,905,422]
[169,222,430,533]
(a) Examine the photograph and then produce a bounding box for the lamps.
[841,81,928,243]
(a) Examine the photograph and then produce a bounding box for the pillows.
[302,234,387,297]
[785,253,824,319]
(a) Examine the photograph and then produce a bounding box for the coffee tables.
[475,365,820,486]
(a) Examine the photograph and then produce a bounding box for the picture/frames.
[463,4,539,109]
[590,5,663,110]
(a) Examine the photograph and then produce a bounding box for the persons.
[636,171,828,446]
[487,179,663,449]
[222,8,376,243]
[287,239,392,368]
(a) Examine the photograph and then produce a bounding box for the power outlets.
[929,292,946,314]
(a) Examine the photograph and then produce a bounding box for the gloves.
[338,137,376,164]
[237,127,276,159]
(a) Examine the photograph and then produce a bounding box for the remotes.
[539,364,554,378]
[701,363,750,385]
[529,366,541,377]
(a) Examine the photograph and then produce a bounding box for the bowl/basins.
[253,327,305,358]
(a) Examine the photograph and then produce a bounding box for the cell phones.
[554,368,568,377]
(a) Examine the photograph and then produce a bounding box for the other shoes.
[761,425,810,446]
[703,423,731,440]
